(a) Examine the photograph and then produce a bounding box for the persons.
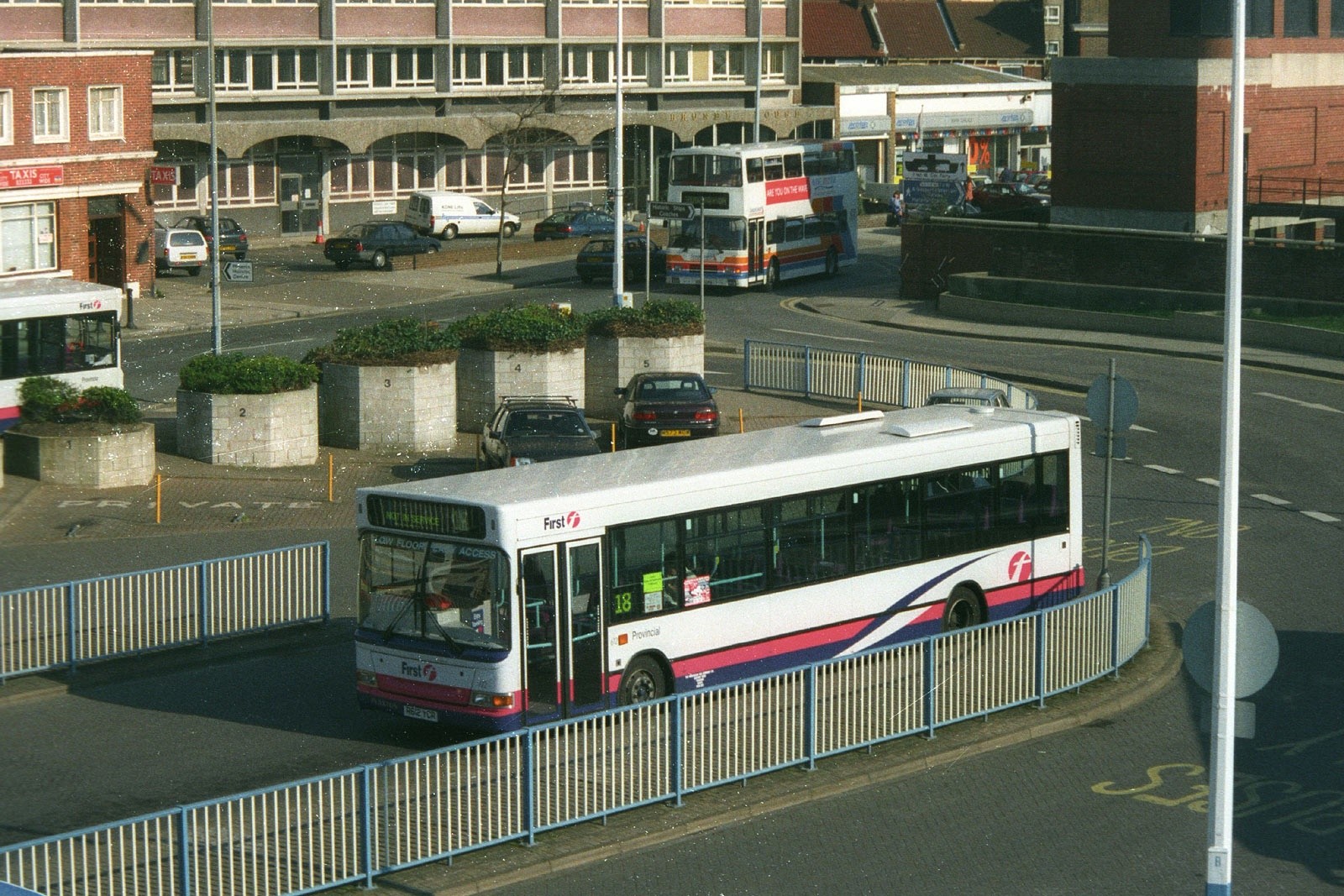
[999,167,1010,182]
[966,176,973,200]
[889,190,902,226]
[664,550,696,594]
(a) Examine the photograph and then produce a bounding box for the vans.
[403,191,522,241]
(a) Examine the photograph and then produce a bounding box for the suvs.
[170,214,249,260]
[153,225,210,277]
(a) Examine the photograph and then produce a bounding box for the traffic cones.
[310,220,326,244]
[639,220,646,232]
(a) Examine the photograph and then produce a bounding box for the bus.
[663,137,858,293]
[350,402,1086,745]
[0,277,124,439]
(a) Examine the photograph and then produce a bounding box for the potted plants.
[307,316,462,448]
[448,301,587,434]
[177,353,321,468]
[582,299,707,414]
[3,378,155,488]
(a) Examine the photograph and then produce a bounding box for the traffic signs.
[649,199,696,222]
[223,261,254,283]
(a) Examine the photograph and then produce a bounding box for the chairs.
[640,382,656,395]
[710,475,1062,599]
[717,162,845,187]
[680,380,696,393]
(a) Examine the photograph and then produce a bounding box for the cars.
[533,200,639,242]
[914,166,1053,219]
[924,385,1012,408]
[575,233,666,284]
[480,393,605,469]
[322,218,443,272]
[614,369,723,451]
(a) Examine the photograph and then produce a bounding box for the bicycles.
[593,188,634,224]
